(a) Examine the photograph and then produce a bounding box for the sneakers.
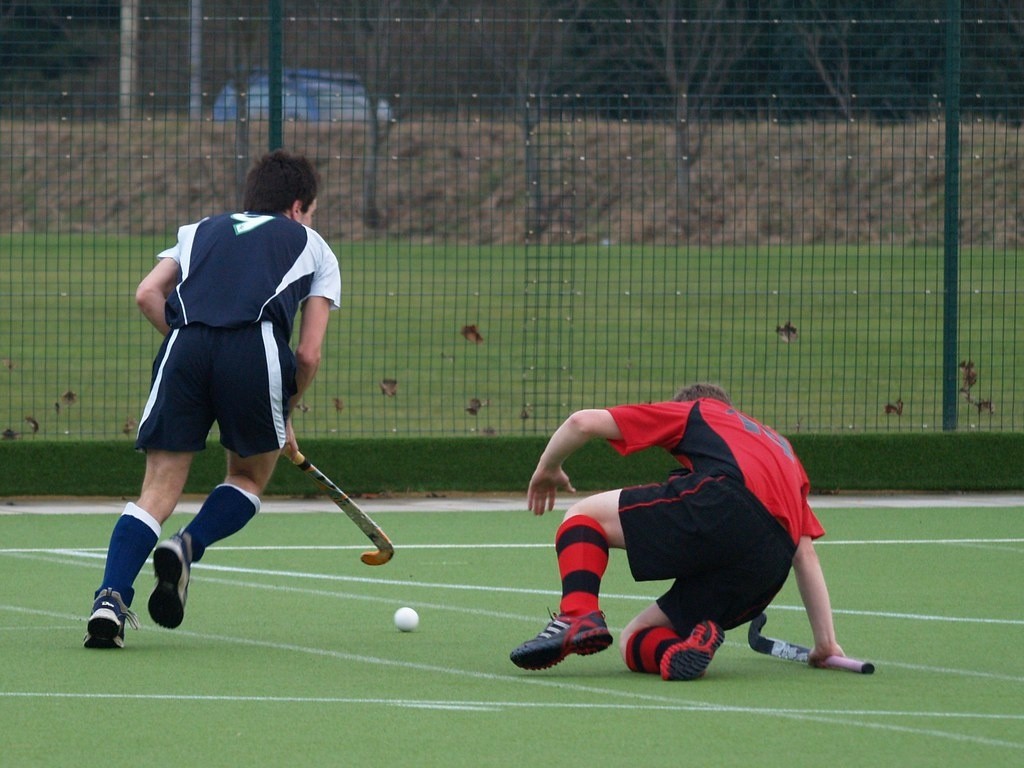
[84,588,140,649]
[510,605,614,670]
[661,620,725,680]
[148,526,190,629]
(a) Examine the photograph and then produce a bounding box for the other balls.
[394,607,420,631]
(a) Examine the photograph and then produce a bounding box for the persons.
[82,153,342,648]
[509,384,847,680]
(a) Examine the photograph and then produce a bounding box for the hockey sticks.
[746,612,875,674]
[278,442,396,567]
